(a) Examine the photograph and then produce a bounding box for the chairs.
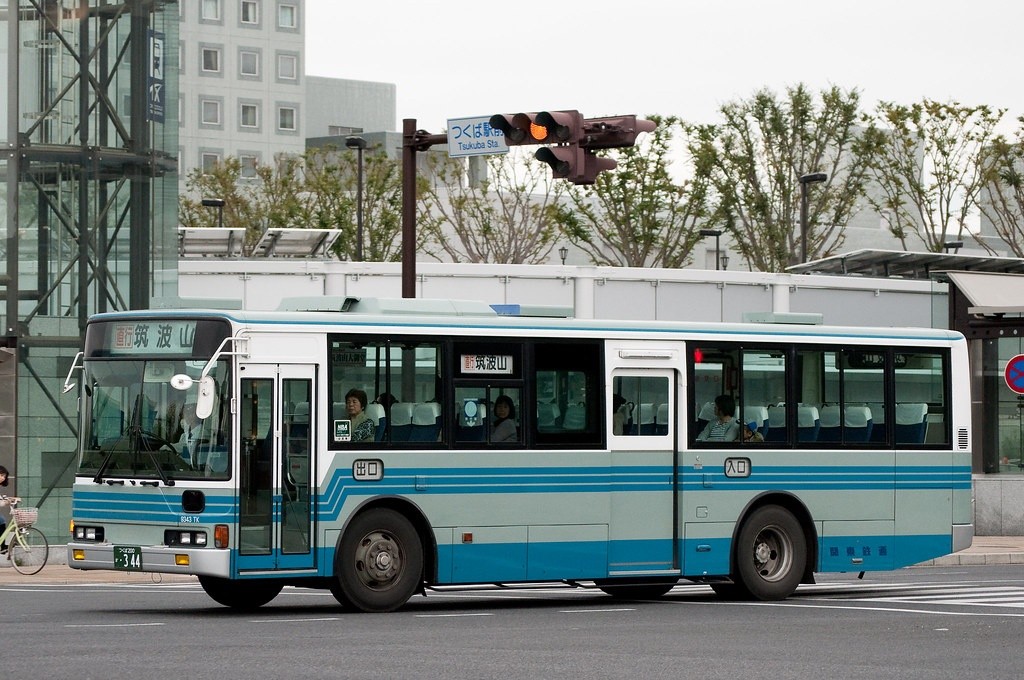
[733,406,740,419]
[332,401,350,419]
[896,402,927,443]
[695,402,701,422]
[631,402,655,435]
[538,402,561,427]
[798,402,804,406]
[562,402,585,429]
[816,405,840,440]
[844,406,873,442]
[293,402,309,423]
[458,403,487,441]
[455,402,462,439]
[775,406,785,440]
[776,401,785,408]
[698,401,717,436]
[744,405,769,440]
[867,402,898,442]
[285,401,295,422]
[408,402,442,441]
[655,403,668,434]
[391,402,413,441]
[798,406,820,441]
[364,402,387,442]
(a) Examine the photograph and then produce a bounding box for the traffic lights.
[575,155,617,186]
[586,114,656,150]
[535,146,585,180]
[487,110,585,143]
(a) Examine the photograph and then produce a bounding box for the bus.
[61,295,976,613]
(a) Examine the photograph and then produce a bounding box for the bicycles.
[0,494,49,575]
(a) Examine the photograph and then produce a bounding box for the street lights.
[199,199,223,225]
[699,229,722,272]
[559,247,568,264]
[798,174,828,265]
[721,255,730,271]
[347,136,368,259]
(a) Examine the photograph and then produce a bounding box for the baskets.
[12,507,38,526]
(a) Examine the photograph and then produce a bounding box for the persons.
[734,417,764,442]
[0,465,21,555]
[340,388,375,442]
[697,394,741,442]
[376,392,399,441]
[157,403,213,471]
[613,393,626,435]
[489,394,518,442]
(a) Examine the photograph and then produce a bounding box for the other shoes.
[0,545,15,553]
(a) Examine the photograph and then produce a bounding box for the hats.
[736,418,758,435]
[184,394,197,405]
